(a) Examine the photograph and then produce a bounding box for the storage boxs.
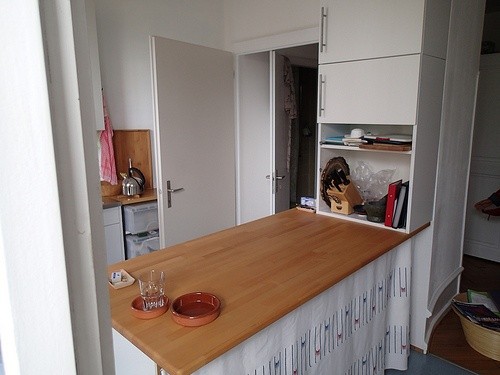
[124,202,159,233]
[125,230,160,259]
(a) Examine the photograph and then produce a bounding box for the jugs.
[121,167,145,196]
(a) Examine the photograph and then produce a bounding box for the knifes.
[321,168,350,192]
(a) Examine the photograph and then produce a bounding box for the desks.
[107,207,431,375]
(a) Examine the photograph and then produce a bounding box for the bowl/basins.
[365,200,387,223]
[101,179,122,197]
[451,291,500,362]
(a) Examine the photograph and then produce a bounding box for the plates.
[320,156,352,207]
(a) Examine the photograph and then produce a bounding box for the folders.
[385,180,402,227]
[392,181,409,229]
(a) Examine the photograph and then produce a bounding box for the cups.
[137,269,166,312]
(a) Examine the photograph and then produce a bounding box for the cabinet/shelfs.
[315,0,453,233]
[103,206,125,265]
